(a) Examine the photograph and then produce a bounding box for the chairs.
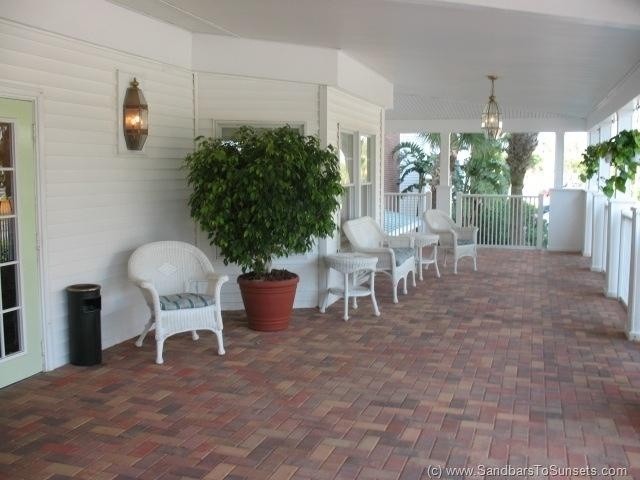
[342,217,416,304]
[126,240,229,365]
[423,209,480,274]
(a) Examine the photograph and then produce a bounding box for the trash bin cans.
[66,284,102,366]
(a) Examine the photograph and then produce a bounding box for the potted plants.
[181,124,346,331]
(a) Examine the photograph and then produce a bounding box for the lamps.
[123,76,149,151]
[481,74,503,140]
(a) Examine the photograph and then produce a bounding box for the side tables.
[398,232,441,282]
[319,252,381,322]
